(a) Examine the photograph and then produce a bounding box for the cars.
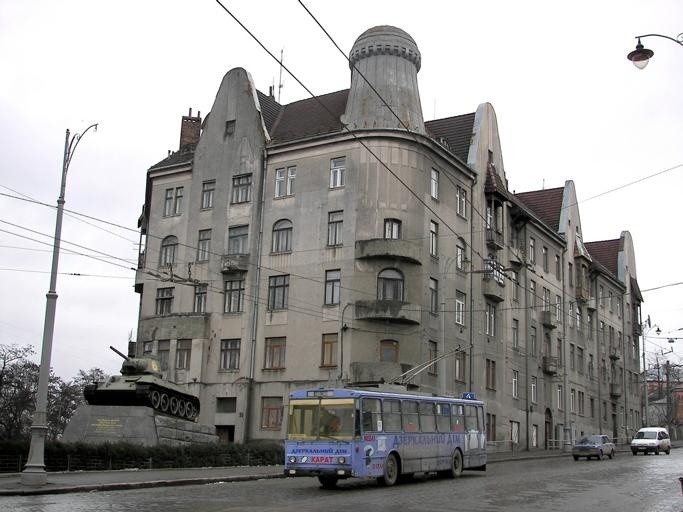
[568,433,616,462]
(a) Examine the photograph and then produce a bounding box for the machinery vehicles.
[81,344,202,422]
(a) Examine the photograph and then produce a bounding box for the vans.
[628,427,671,457]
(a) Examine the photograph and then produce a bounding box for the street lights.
[625,30,683,71]
[640,322,661,427]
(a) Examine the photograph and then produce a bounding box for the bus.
[279,387,488,491]
